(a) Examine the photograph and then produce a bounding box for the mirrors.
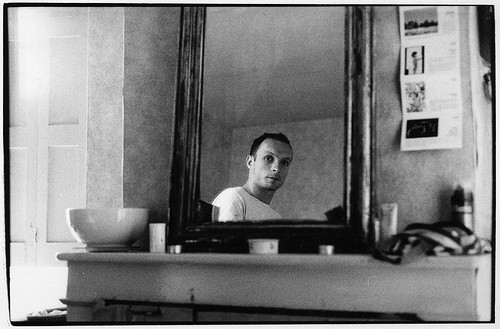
[169,6,378,253]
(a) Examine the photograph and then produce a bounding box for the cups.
[149,223,166,252]
[382,204,398,241]
[167,245,182,254]
[451,188,474,233]
[318,245,333,256]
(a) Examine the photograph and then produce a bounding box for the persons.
[210,132,293,223]
[411,51,423,75]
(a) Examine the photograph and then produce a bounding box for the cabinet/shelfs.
[59,252,493,320]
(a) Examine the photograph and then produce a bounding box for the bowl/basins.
[249,239,279,255]
[67,208,149,252]
